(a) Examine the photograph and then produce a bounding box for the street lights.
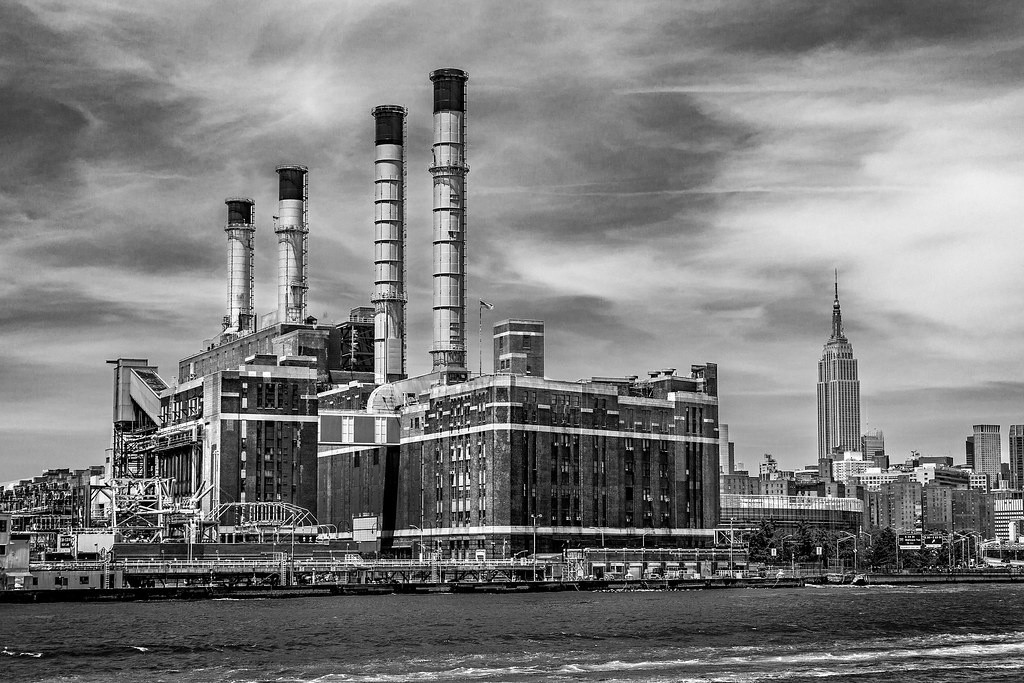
[859,531,873,574]
[781,534,793,563]
[531,514,543,581]
[642,530,652,562]
[409,524,423,566]
[836,534,856,574]
[886,524,903,573]
[589,527,604,548]
[840,530,857,573]
